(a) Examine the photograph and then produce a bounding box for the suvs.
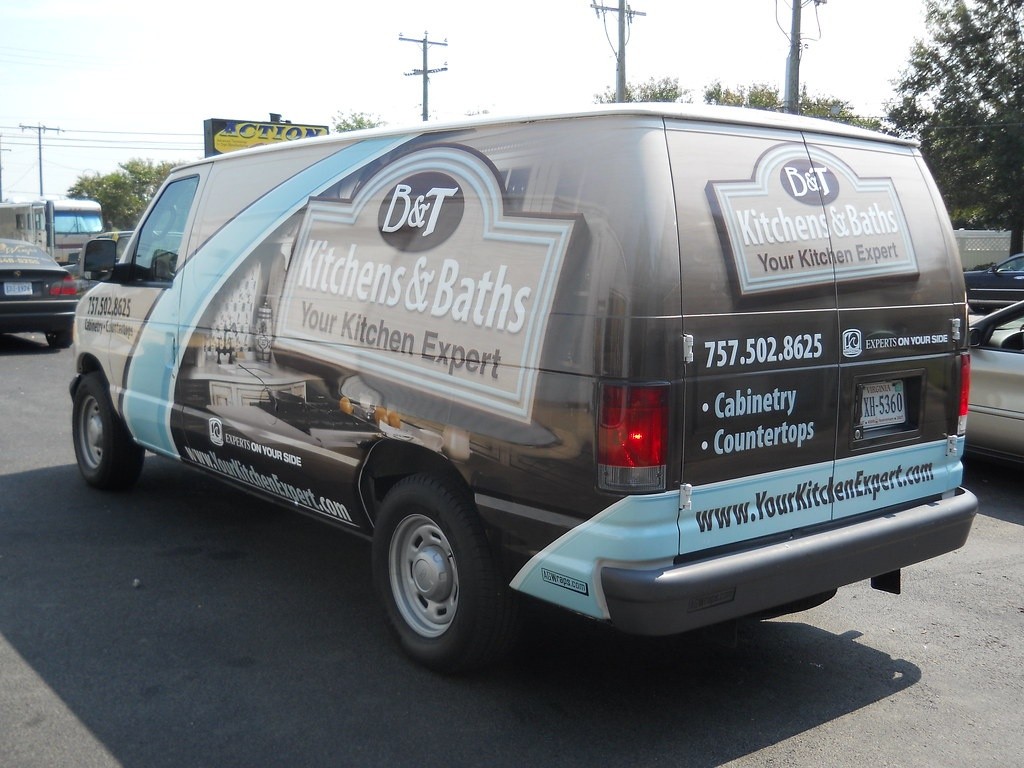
[62,230,187,301]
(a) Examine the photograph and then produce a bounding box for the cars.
[959,298,1024,470]
[964,253,1024,300]
[0,239,77,348]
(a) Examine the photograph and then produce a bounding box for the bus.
[0,196,106,268]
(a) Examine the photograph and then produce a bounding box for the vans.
[69,103,979,671]
